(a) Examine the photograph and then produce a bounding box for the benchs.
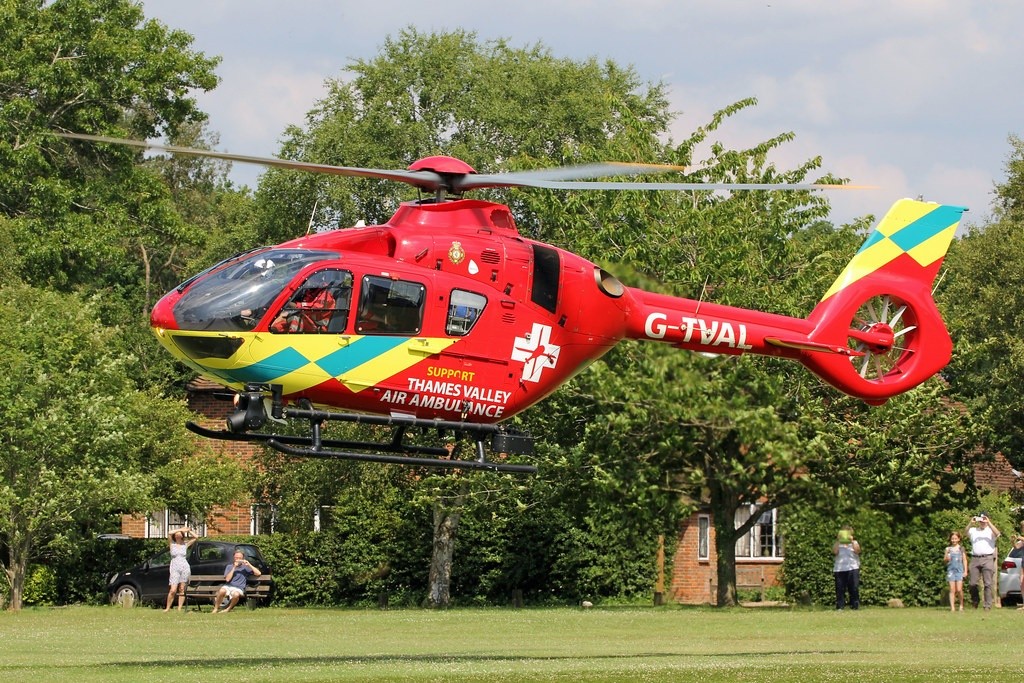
[187,575,271,610]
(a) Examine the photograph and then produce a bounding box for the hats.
[979,512,989,518]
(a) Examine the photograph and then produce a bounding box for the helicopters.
[32,130,971,476]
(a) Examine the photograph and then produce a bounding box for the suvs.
[107,537,273,610]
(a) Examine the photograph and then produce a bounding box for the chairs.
[209,552,217,560]
[320,286,346,332]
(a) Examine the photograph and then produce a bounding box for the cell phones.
[975,517,983,521]
[236,560,244,565]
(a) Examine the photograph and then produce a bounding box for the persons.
[163,527,198,612]
[241,258,335,334]
[964,512,1000,612]
[944,531,968,612]
[1011,521,1024,611]
[211,549,261,614]
[832,525,861,609]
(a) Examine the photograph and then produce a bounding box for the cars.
[996,539,1024,608]
